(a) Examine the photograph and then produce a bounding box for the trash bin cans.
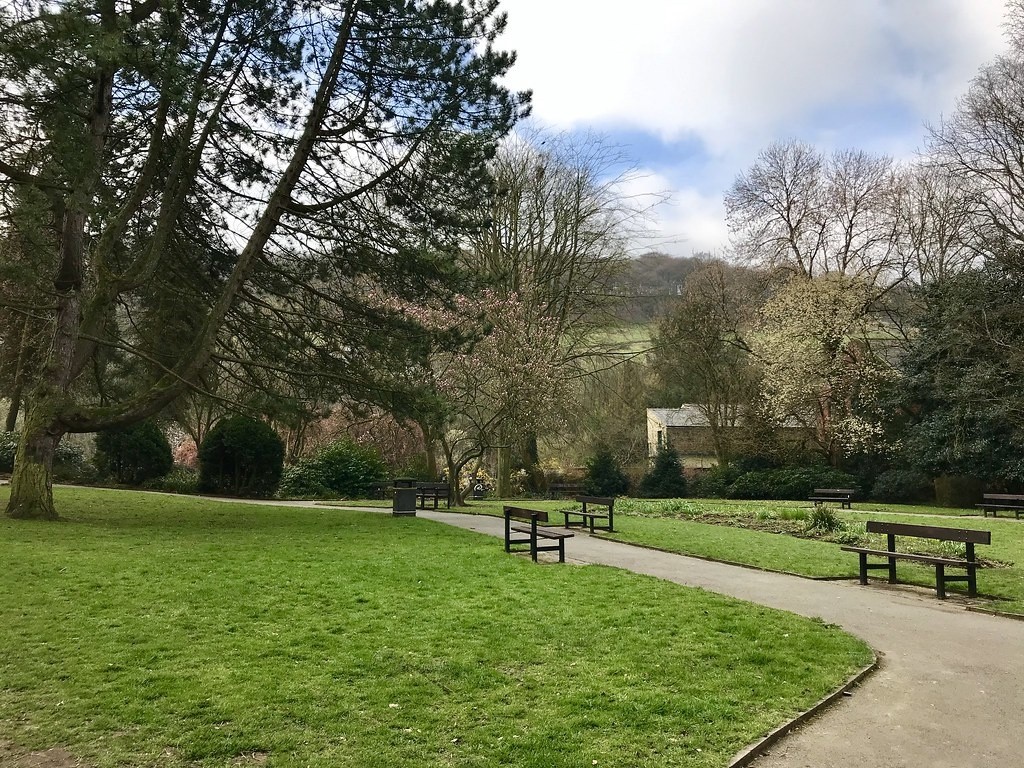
[469,477,484,501]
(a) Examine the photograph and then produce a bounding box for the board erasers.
[391,476,419,517]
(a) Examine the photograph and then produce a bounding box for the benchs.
[560,496,615,533]
[841,521,992,599]
[415,482,451,511]
[502,506,573,562]
[975,494,1024,520]
[808,489,855,509]
